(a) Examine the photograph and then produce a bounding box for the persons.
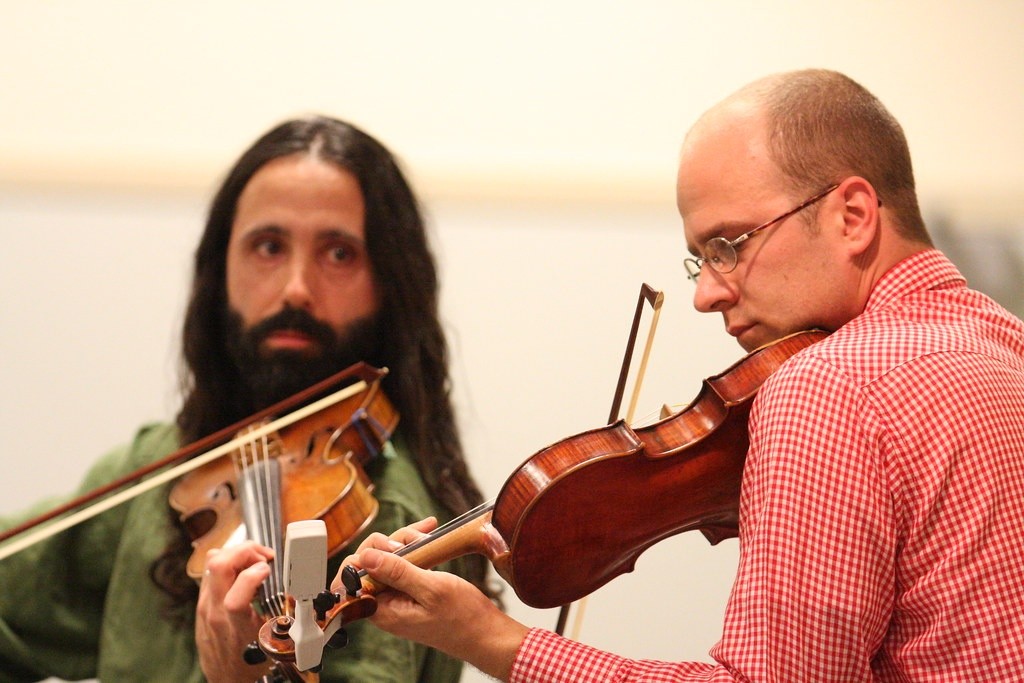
[0,116,494,683]
[331,67,1023,683]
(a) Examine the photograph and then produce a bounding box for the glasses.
[683,185,882,288]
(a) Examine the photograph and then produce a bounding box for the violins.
[241,323,833,682]
[163,377,407,683]
